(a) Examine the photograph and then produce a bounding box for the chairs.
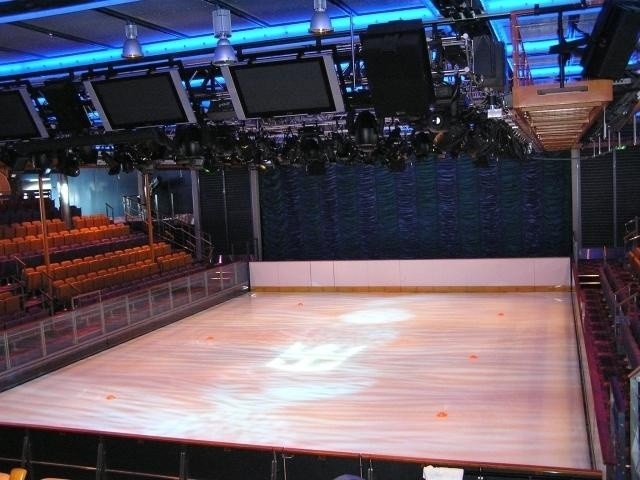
[0,197,208,329]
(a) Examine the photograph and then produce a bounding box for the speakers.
[359,21,435,118]
[483,47,505,87]
[40,80,91,131]
[580,0,640,80]
[473,35,496,77]
[444,45,467,70]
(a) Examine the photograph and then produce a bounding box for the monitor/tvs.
[91,72,189,130]
[0,90,41,141]
[229,56,336,118]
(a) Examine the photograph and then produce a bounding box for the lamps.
[22,108,534,179]
[119,0,336,70]
